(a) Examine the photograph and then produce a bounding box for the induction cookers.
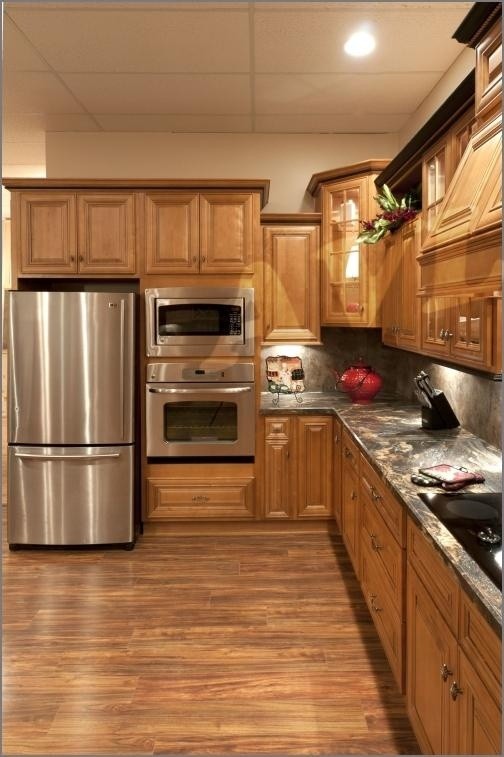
[416,493,501,595]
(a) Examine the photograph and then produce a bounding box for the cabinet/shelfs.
[11,189,136,277]
[422,1,503,380]
[142,462,261,534]
[142,189,260,278]
[405,514,503,757]
[381,211,421,353]
[259,414,333,520]
[259,212,319,345]
[333,418,404,693]
[321,159,392,327]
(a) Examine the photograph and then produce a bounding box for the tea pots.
[334,355,383,404]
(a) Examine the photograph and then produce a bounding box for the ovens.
[146,363,254,463]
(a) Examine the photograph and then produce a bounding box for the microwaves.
[144,286,254,357]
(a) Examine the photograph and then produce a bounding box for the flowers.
[354,183,422,243]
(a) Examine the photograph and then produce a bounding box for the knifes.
[408,371,437,409]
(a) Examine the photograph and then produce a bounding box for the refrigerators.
[4,289,139,548]
